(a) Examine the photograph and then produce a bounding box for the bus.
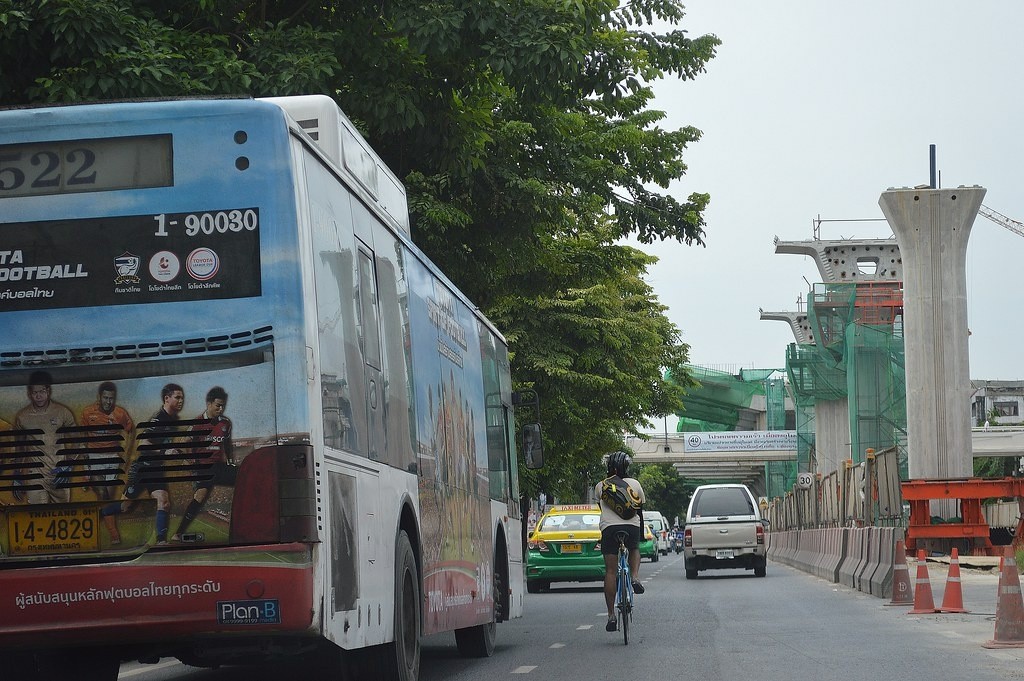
[0,95,546,681]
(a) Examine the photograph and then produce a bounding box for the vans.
[679,483,771,577]
[642,511,669,556]
[664,516,684,553]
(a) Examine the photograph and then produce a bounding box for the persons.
[116,382,191,549]
[595,451,645,632]
[983,419,990,433]
[2,381,79,511]
[76,381,135,556]
[169,386,243,548]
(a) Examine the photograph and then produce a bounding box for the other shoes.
[156,540,170,545]
[110,535,121,545]
[631,577,645,594]
[171,533,182,541]
[605,614,617,631]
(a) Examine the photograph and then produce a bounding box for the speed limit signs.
[688,435,702,448]
[798,472,813,489]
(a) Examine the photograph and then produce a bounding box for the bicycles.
[616,530,640,642]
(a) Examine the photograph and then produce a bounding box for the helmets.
[607,452,632,476]
[649,524,654,529]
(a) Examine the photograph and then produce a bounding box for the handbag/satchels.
[600,475,642,520]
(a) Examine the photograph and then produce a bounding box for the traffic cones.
[934,547,972,613]
[982,545,1024,650]
[908,550,940,614]
[883,539,915,606]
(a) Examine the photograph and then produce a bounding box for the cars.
[639,525,661,563]
[527,504,611,592]
